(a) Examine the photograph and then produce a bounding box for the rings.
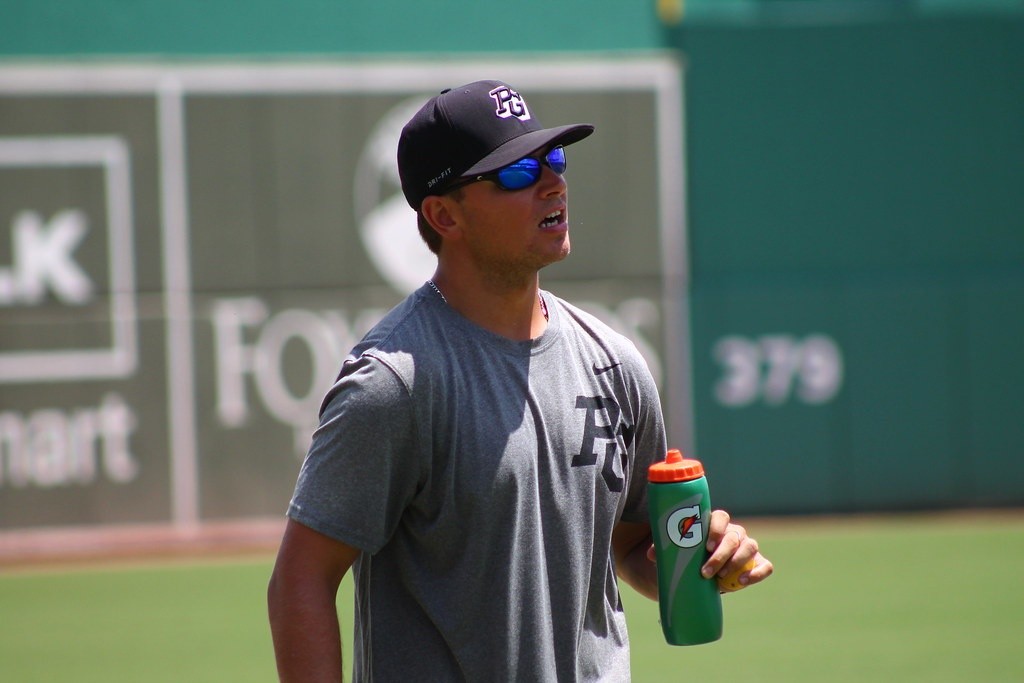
[725,529,740,547]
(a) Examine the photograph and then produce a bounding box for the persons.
[266,79,773,683]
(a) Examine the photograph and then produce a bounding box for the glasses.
[437,143,567,191]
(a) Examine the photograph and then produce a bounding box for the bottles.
[648,449,723,646]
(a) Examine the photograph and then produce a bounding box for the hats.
[396,79,594,214]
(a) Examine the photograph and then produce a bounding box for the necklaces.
[428,278,549,322]
